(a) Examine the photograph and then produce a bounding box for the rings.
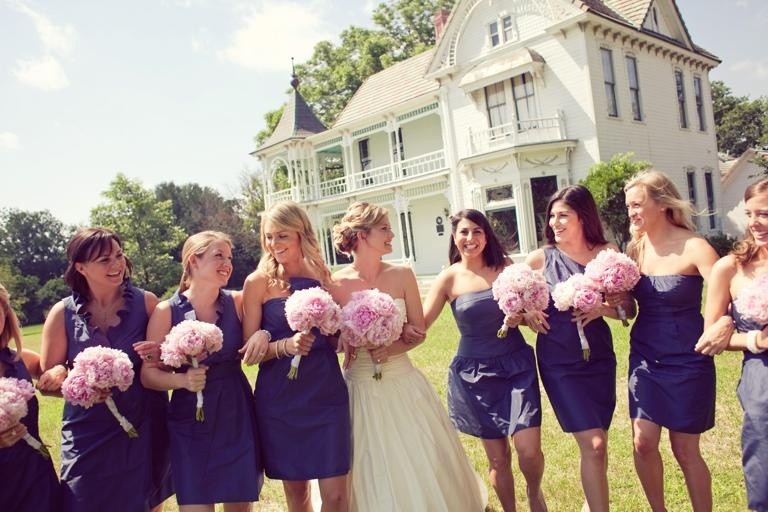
[376,358,380,362]
[147,354,152,362]
[259,352,263,355]
[12,430,15,434]
[534,319,541,325]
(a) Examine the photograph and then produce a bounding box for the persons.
[30,225,173,512]
[0,284,69,512]
[523,185,630,512]
[238,201,353,512]
[605,170,734,512]
[402,208,558,512]
[327,199,488,512]
[703,177,768,512]
[140,231,275,512]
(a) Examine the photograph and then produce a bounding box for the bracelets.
[275,339,280,359]
[258,330,281,339]
[745,327,763,358]
[283,339,289,359]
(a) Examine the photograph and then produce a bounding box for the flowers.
[60,344,139,440]
[283,285,402,383]
[1,376,53,465]
[731,272,768,327]
[491,247,642,364]
[159,318,224,424]
